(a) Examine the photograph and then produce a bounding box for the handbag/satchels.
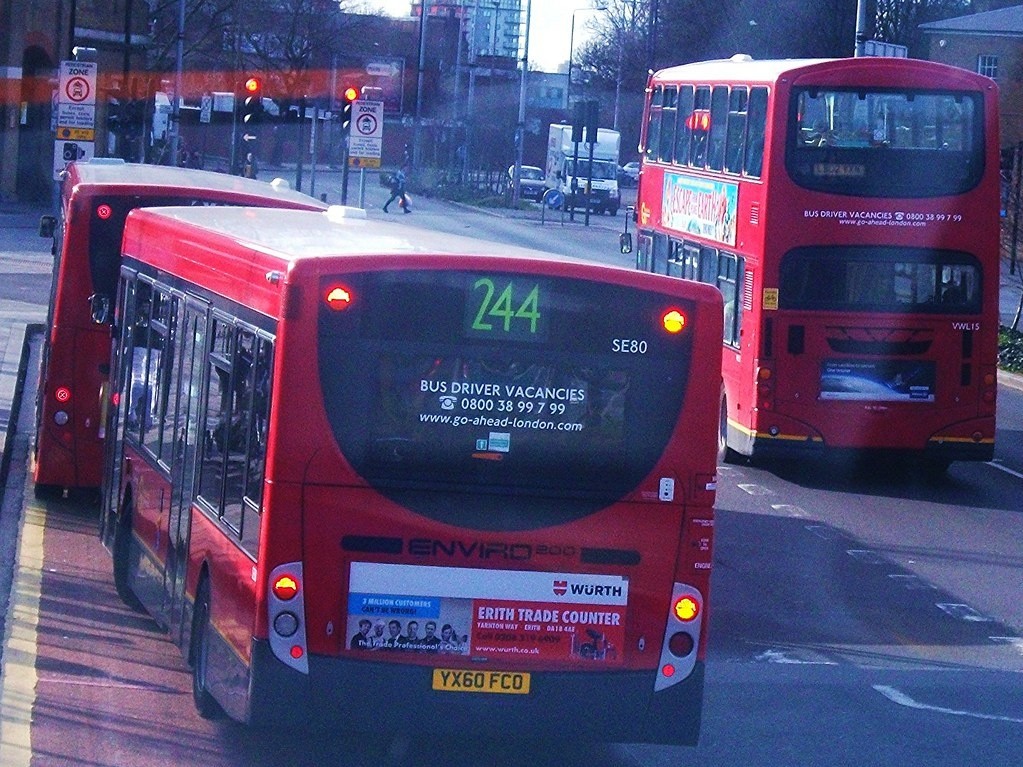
[399,193,413,207]
[380,173,400,189]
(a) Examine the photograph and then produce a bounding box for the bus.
[620,52,1000,480]
[98,205,725,749]
[32,156,329,499]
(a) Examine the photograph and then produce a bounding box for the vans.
[506,165,545,203]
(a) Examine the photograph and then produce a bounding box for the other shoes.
[404,209,411,213]
[383,207,388,213]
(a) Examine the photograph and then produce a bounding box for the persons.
[405,622,421,647]
[368,620,388,648]
[381,163,412,216]
[351,620,372,648]
[150,131,260,181]
[438,623,461,654]
[419,621,442,651]
[384,620,405,647]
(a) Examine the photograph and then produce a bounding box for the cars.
[617,165,635,187]
[623,162,640,182]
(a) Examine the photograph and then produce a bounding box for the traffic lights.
[586,102,600,142]
[341,86,362,136]
[571,101,587,142]
[240,75,261,124]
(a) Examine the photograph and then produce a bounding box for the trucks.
[546,124,621,216]
